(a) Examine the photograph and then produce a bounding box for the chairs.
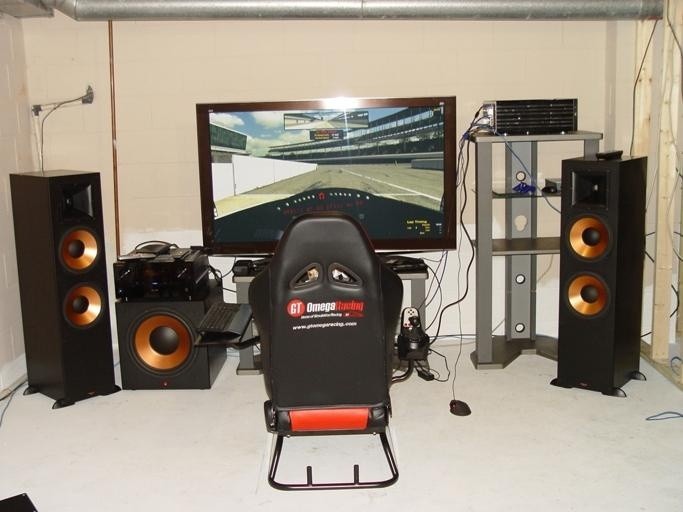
[248,211,403,489]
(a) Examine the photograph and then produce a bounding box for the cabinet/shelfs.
[467,130,604,370]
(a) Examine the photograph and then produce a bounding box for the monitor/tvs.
[194,95,459,256]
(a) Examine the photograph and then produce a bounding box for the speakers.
[8,168,121,409]
[548,154,647,397]
[115,278,228,390]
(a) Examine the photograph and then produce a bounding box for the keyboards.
[196,301,252,339]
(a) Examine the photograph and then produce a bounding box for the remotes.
[117,253,158,261]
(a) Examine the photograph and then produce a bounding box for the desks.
[232,269,429,375]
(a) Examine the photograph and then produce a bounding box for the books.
[118,244,168,261]
